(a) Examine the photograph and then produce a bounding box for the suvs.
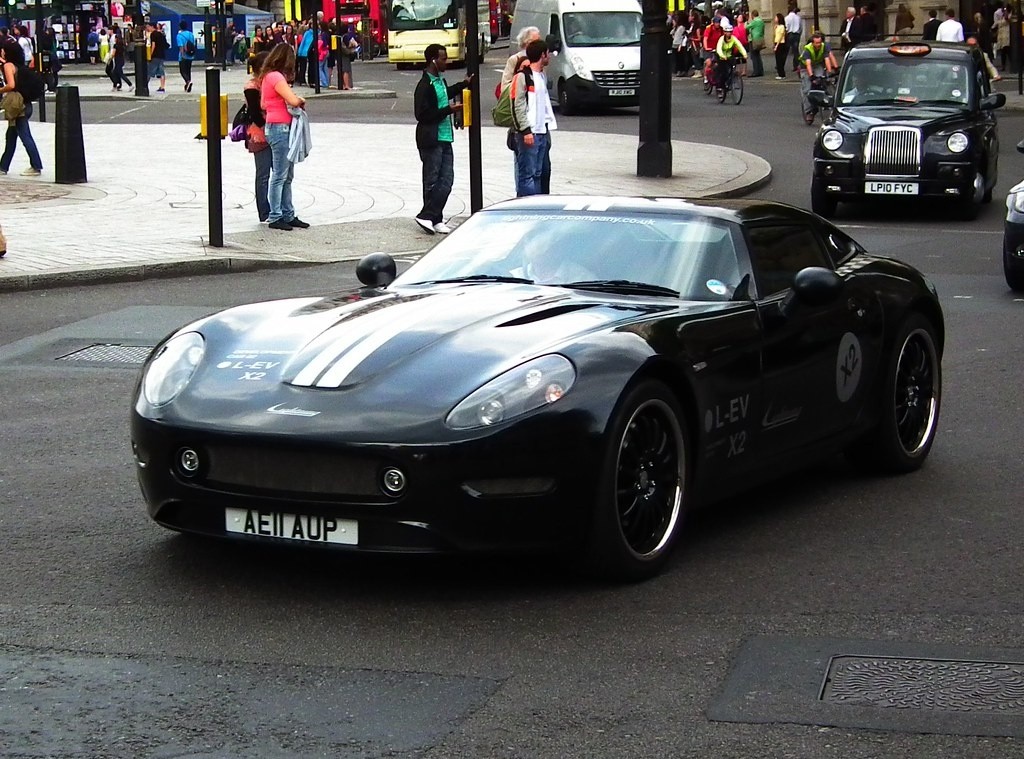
[808,40,1007,222]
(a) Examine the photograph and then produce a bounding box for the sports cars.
[128,195,946,584]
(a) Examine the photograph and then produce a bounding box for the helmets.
[723,24,733,32]
[711,17,721,22]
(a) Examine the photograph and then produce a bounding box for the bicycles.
[800,66,843,127]
[704,48,747,106]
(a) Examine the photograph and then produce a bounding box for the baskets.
[735,63,747,77]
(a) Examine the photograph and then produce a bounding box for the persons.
[176,21,195,92]
[894,3,915,40]
[839,5,876,55]
[87,23,138,92]
[244,42,310,232]
[509,227,599,286]
[922,10,942,40]
[936,8,964,42]
[666,3,765,98]
[798,31,841,121]
[226,16,362,91]
[615,24,628,38]
[845,73,883,96]
[414,44,474,234]
[0,24,63,175]
[973,1,1013,71]
[967,38,1002,86]
[143,22,167,92]
[773,3,803,79]
[500,25,558,197]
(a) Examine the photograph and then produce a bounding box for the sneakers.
[414,215,436,235]
[433,222,451,234]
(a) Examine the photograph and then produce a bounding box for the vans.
[508,0,643,116]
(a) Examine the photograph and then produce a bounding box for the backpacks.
[179,33,195,56]
[2,62,44,102]
[491,71,531,127]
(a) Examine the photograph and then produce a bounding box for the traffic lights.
[224,0,234,19]
[330,34,338,51]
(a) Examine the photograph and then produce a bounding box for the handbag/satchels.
[229,104,269,153]
[153,32,170,51]
[341,34,359,55]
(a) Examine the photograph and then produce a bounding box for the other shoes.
[0,170,7,175]
[288,216,310,229]
[156,87,165,93]
[676,70,785,91]
[20,168,41,176]
[45,89,54,95]
[262,218,270,224]
[290,81,352,90]
[184,82,192,93]
[110,87,122,92]
[806,113,813,122]
[128,85,134,92]
[269,219,293,231]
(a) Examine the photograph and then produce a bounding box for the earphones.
[433,59,436,64]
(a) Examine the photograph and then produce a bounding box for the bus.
[388,0,491,67]
